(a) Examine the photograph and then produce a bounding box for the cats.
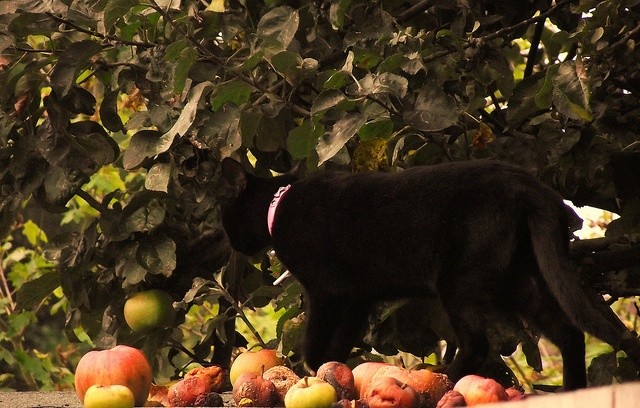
[219,154,636,391]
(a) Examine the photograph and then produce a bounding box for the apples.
[233,365,277,407]
[124,289,177,335]
[284,376,337,408]
[453,374,484,400]
[466,378,509,407]
[74,344,152,407]
[82,385,135,408]
[397,367,456,408]
[263,366,301,408]
[349,362,391,400]
[316,361,355,400]
[229,349,285,385]
[367,378,417,408]
